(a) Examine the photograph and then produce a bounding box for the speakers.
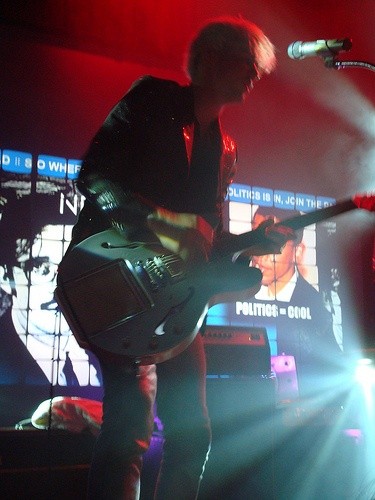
[205,375,279,466]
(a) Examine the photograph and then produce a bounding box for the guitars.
[54,188,375,366]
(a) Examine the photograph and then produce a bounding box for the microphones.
[288,38,352,61]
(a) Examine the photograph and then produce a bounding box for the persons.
[247,204,319,301]
[53,15,276,500]
